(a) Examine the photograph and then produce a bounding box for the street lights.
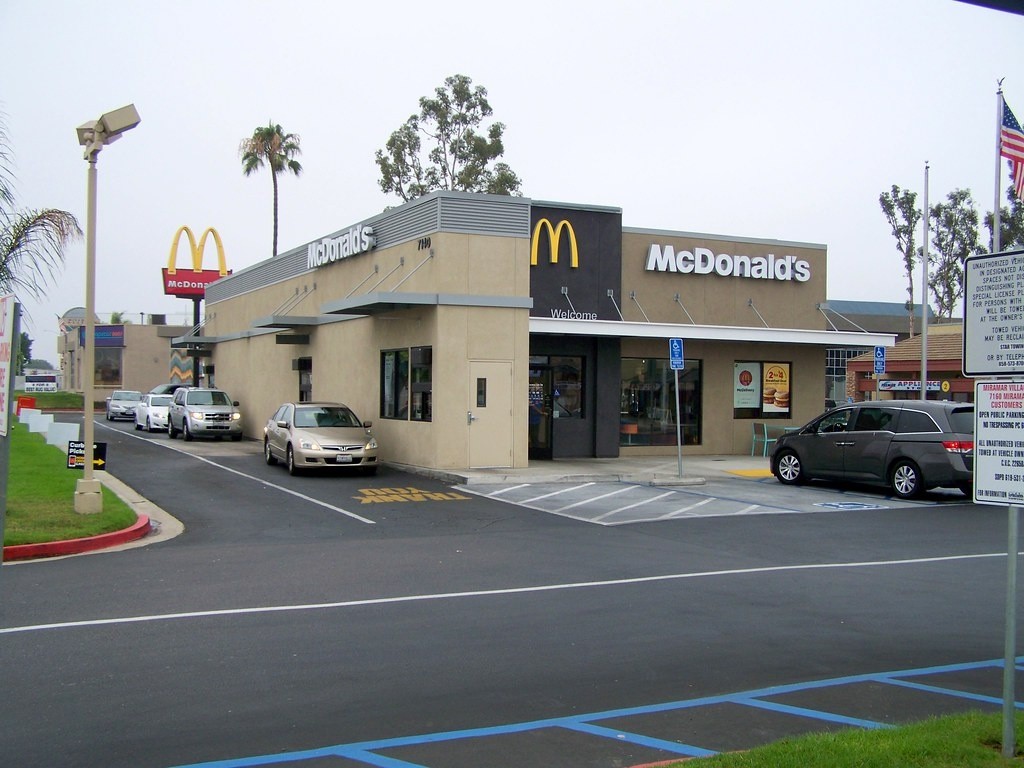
[73,103,141,514]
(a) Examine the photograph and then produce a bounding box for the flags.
[57,316,67,332]
[1000,92,1024,203]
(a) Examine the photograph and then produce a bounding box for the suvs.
[167,385,243,441]
[770,400,975,500]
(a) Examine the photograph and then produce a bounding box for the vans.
[146,383,193,396]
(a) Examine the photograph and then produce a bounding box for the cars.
[262,400,379,475]
[134,394,174,432]
[106,390,144,421]
